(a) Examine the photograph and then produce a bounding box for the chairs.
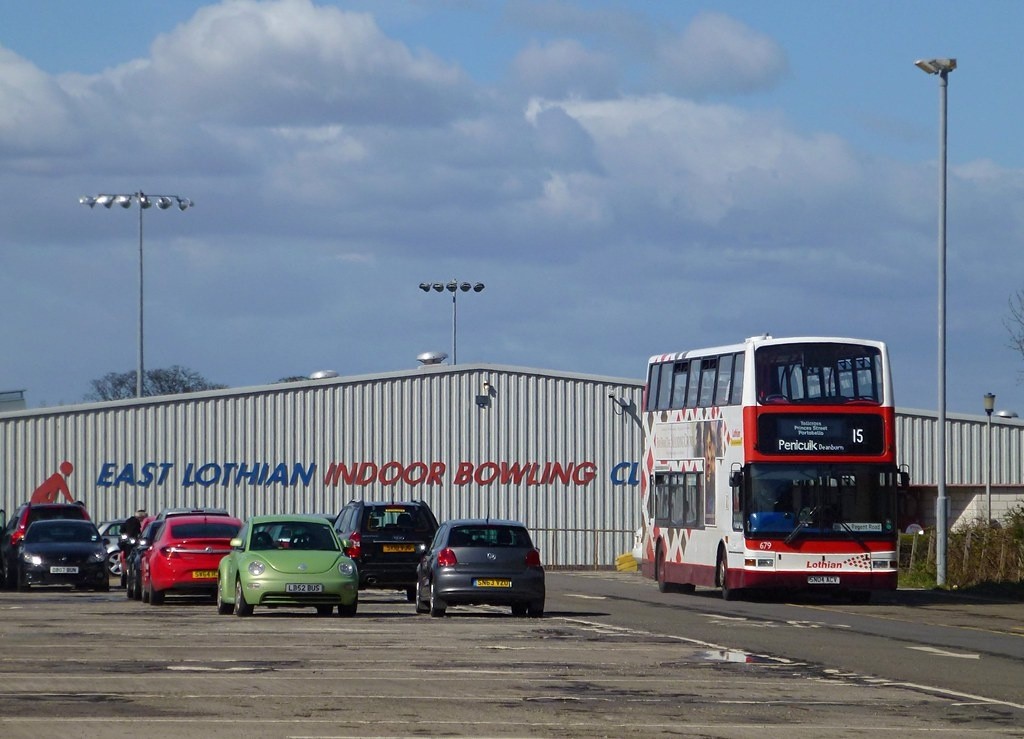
[452,527,516,548]
[370,513,415,532]
[249,532,335,551]
[36,529,96,545]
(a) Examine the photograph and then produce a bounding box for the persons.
[677,501,691,527]
[116,509,147,588]
[73,500,85,508]
[693,420,724,526]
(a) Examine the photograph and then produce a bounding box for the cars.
[0,500,230,599]
[410,518,547,616]
[141,513,250,603]
[18,519,111,594]
[219,516,359,619]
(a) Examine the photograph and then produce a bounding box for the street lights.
[982,391,1002,517]
[420,277,487,369]
[77,189,191,396]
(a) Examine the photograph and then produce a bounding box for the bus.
[642,335,912,602]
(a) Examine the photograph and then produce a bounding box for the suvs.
[332,497,441,595]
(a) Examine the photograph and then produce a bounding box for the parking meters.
[908,54,957,589]
